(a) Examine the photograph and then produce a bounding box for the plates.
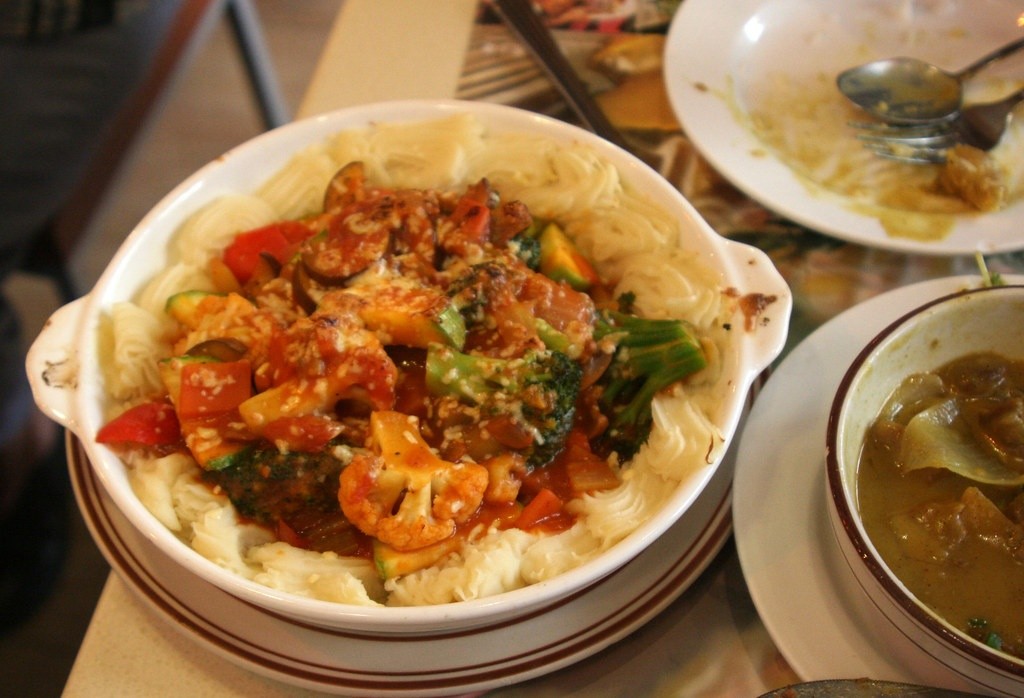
[733,273,1024,698]
[63,367,762,698]
[664,0,1024,257]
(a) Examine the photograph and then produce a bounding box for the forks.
[849,89,1024,167]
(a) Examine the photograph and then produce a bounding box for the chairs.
[0,0,294,338]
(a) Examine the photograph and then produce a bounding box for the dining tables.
[60,1,1024,698]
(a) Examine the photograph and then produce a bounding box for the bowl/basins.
[827,285,1024,698]
[23,98,795,637]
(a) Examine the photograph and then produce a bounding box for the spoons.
[836,36,1024,120]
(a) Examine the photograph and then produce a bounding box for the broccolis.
[421,339,584,469]
[225,265,490,553]
[587,308,706,454]
